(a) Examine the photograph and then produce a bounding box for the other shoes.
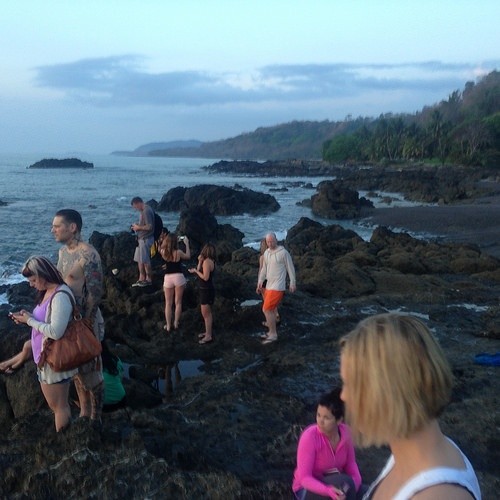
[198,338,213,345]
[198,333,205,337]
[259,333,277,345]
[262,322,269,327]
[132,280,152,287]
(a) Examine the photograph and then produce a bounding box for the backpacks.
[152,212,163,241]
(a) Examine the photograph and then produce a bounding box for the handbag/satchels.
[37,290,102,373]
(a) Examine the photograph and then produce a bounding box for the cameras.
[179,236,185,240]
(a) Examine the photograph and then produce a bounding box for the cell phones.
[10,313,15,317]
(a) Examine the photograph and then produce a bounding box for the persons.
[187,243,217,347]
[339,310,483,500]
[1,208,126,420]
[292,386,362,500]
[8,255,84,433]
[159,233,191,334]
[256,232,296,345]
[128,195,171,288]
